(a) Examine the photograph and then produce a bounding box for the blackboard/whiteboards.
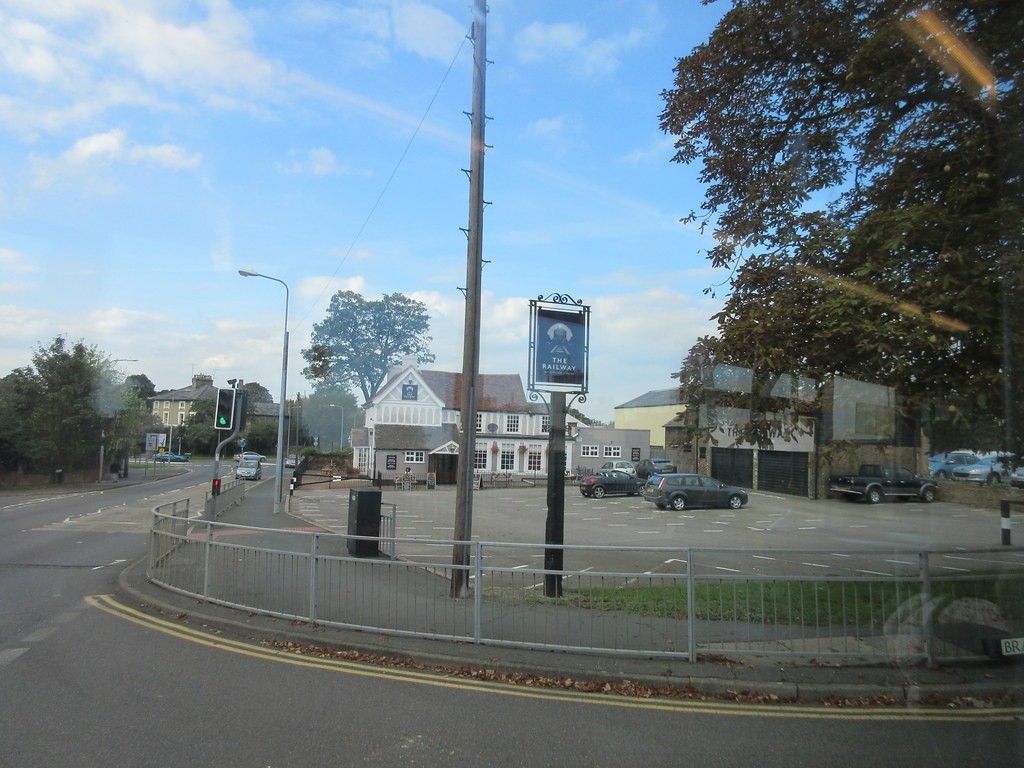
[402,474,410,488]
[427,472,436,487]
[473,473,482,487]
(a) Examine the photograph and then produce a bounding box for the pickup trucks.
[828,463,941,504]
[233,451,267,464]
[580,468,650,499]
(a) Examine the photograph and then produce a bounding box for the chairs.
[395,473,417,491]
[691,479,696,485]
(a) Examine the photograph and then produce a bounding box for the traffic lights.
[215,387,235,430]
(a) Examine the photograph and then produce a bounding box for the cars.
[601,460,639,483]
[952,454,1024,489]
[236,460,262,481]
[637,456,679,481]
[152,450,190,462]
[285,454,303,469]
[930,453,981,484]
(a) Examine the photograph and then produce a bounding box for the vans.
[644,472,748,512]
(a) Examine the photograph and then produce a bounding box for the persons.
[638,462,647,488]
[110,460,121,482]
[404,468,412,487]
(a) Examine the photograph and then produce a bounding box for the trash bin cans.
[348,487,382,557]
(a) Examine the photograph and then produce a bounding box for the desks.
[318,468,333,479]
[326,465,338,474]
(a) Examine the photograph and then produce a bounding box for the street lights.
[329,404,344,452]
[97,357,140,483]
[239,268,289,516]
